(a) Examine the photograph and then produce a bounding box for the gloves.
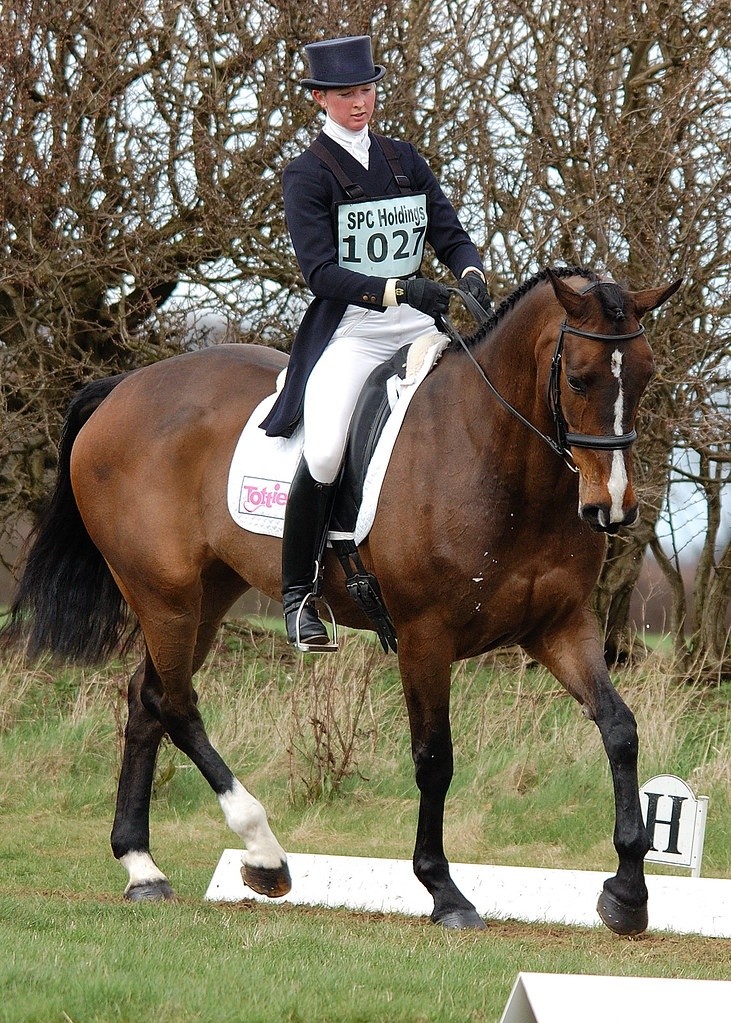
[395,277,450,318]
[457,270,491,311]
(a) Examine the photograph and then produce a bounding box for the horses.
[0,265,686,937]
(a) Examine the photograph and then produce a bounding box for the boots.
[280,451,331,646]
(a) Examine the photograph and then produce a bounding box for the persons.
[255,35,494,647]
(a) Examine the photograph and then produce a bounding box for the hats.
[299,35,386,87]
